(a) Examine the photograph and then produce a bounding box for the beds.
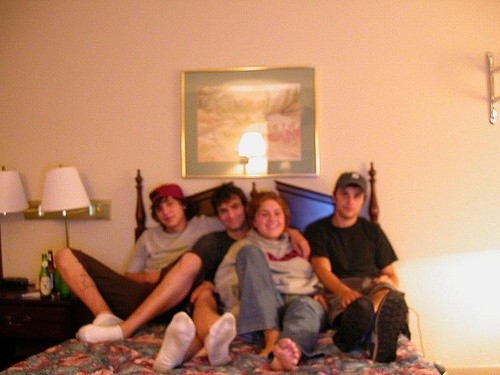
[0,162,447,375]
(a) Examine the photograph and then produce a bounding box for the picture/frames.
[178,64,320,179]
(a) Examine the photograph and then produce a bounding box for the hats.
[336,172,367,197]
[151,186,184,206]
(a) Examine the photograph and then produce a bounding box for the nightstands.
[0,287,91,371]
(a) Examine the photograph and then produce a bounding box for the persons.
[303,169,410,365]
[152,180,329,373]
[53,182,312,345]
[212,189,329,374]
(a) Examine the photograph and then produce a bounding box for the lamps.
[0,171,30,215]
[41,167,91,248]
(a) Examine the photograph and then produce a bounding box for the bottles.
[55,270,70,300]
[48,250,57,294]
[39,254,53,299]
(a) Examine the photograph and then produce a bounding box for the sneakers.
[369,291,406,363]
[333,297,374,353]
